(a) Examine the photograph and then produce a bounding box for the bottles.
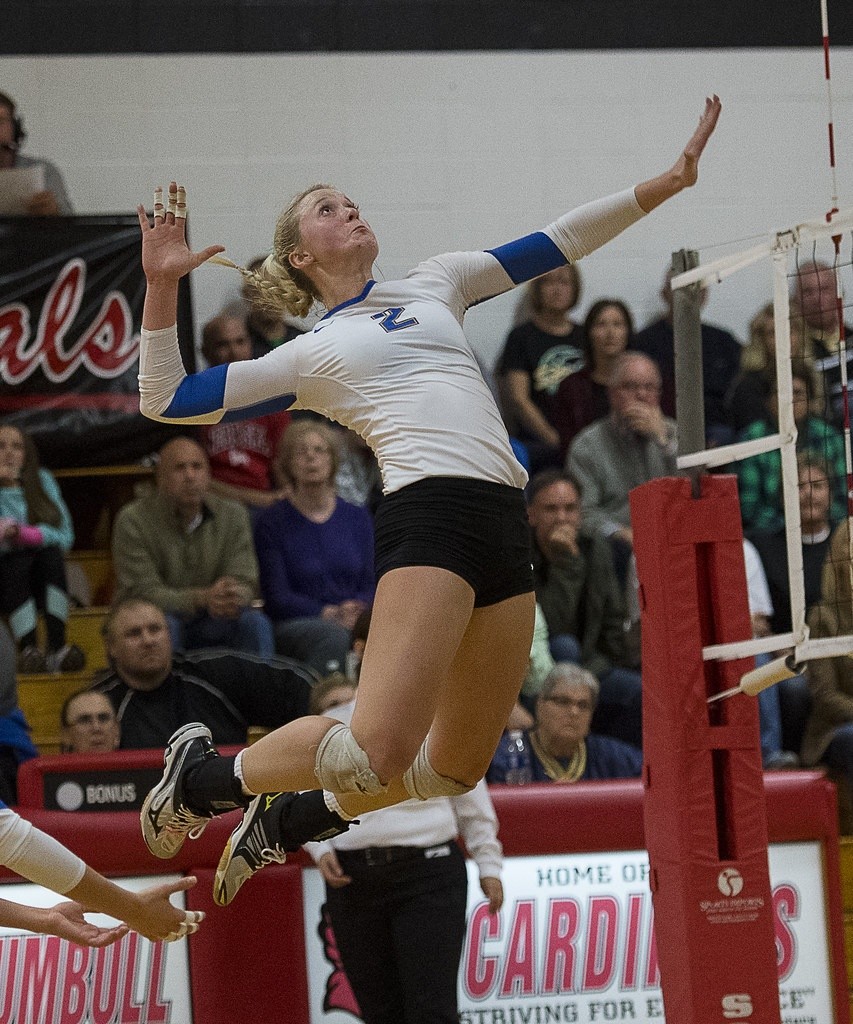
[505,730,532,785]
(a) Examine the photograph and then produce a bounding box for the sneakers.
[140,722,222,859]
[213,791,298,906]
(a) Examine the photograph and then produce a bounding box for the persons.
[0,798,206,948]
[139,93,721,902]
[0,91,74,214]
[0,257,853,835]
[295,609,503,1024]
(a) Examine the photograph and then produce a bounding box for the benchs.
[12,458,153,754]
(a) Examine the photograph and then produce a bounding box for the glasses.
[542,693,593,710]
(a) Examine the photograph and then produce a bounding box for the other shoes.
[48,638,85,671]
[18,645,47,673]
